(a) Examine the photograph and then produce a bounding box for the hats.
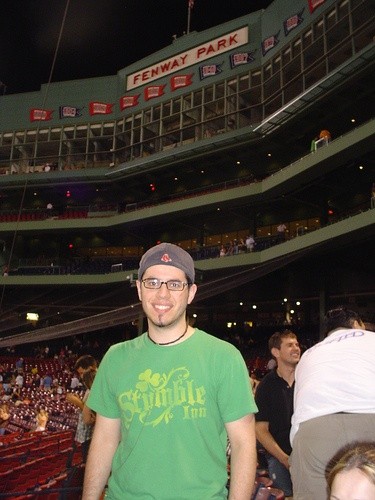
[138,243,195,284]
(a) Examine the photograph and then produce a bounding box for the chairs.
[0,428,87,500]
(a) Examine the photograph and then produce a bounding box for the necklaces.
[147,320,189,346]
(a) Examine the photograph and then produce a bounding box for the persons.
[47,201,53,216]
[1,222,375,500]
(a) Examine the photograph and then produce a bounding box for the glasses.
[141,278,188,291]
